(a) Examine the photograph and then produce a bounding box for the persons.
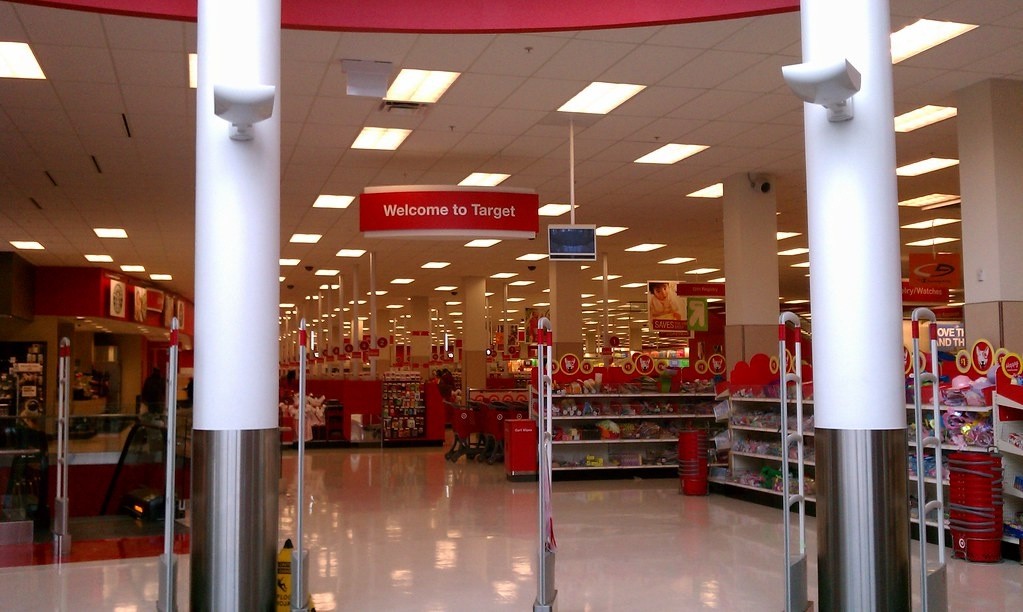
[279,370,296,406]
[527,309,543,329]
[183,377,193,408]
[140,368,166,413]
[649,283,685,321]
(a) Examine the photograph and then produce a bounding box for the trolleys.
[443,398,529,465]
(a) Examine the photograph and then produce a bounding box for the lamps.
[214,83,274,141]
[781,57,861,121]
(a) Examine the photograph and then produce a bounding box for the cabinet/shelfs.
[279,333,1022,563]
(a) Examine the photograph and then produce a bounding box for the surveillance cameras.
[754,179,771,193]
[452,292,457,295]
[305,266,313,271]
[287,285,294,289]
[528,265,536,270]
